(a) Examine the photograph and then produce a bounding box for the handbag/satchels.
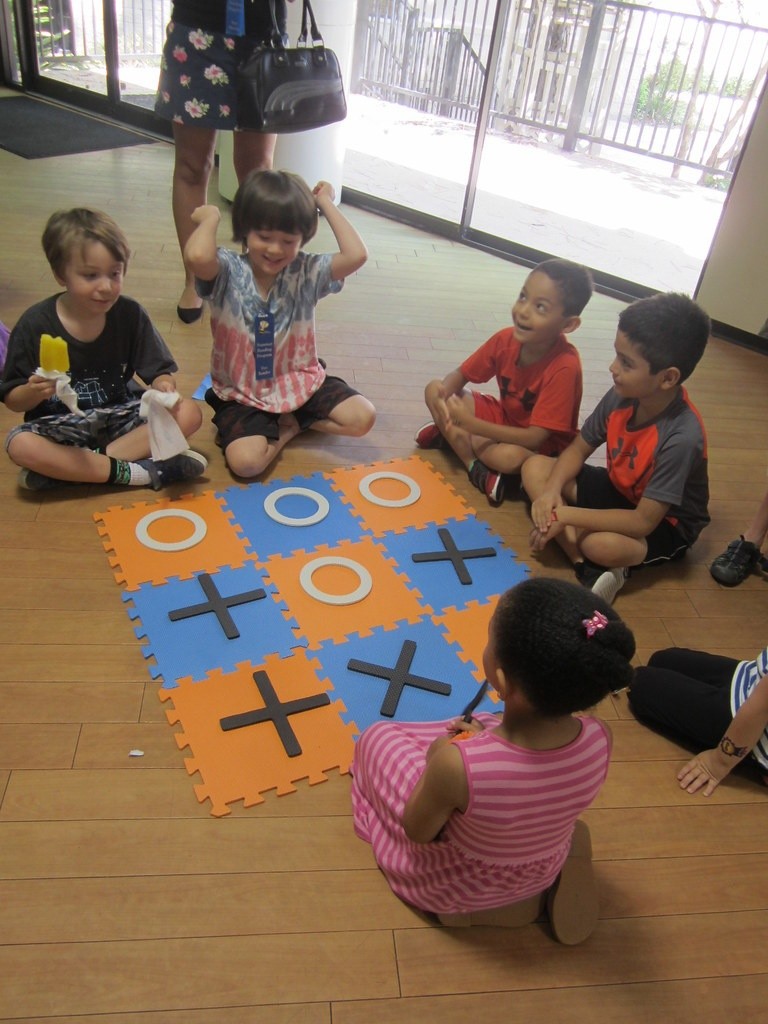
[237,0,348,134]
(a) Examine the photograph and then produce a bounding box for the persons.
[415,257,594,505]
[628,646,768,796]
[710,488,768,587]
[0,207,208,491]
[350,578,635,947]
[521,291,711,606]
[154,0,296,324]
[183,168,377,477]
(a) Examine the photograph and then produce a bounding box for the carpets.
[0,94,162,161]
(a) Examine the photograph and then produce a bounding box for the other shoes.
[178,304,203,324]
[547,820,599,945]
[438,889,545,927]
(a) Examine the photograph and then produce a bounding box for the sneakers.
[415,422,450,449]
[18,467,90,491]
[711,534,760,586]
[468,459,508,503]
[132,450,208,491]
[575,558,628,606]
